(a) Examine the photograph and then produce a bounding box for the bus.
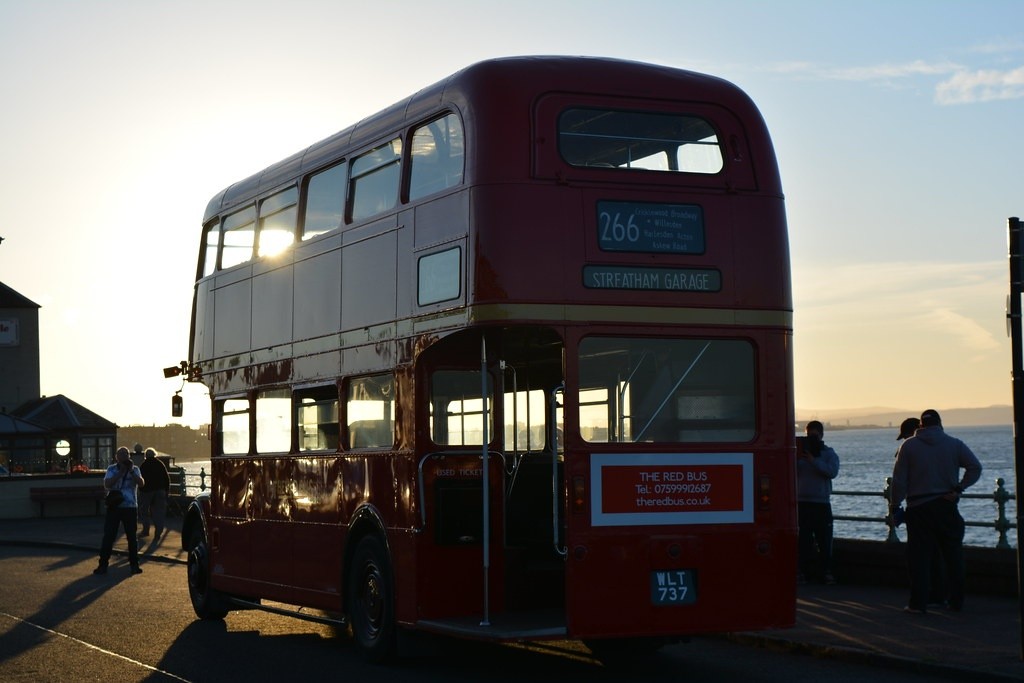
[164,56,800,667]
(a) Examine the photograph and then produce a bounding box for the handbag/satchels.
[105,491,124,509]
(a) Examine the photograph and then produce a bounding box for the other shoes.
[131,562,143,574]
[904,600,929,614]
[93,560,108,575]
[137,532,149,538]
[942,599,962,612]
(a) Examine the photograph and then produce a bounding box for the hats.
[898,418,921,440]
[920,410,943,429]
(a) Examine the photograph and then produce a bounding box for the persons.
[93,447,145,574]
[140,448,170,541]
[796,421,840,596]
[889,409,982,613]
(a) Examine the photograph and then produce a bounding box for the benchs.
[31,484,104,519]
